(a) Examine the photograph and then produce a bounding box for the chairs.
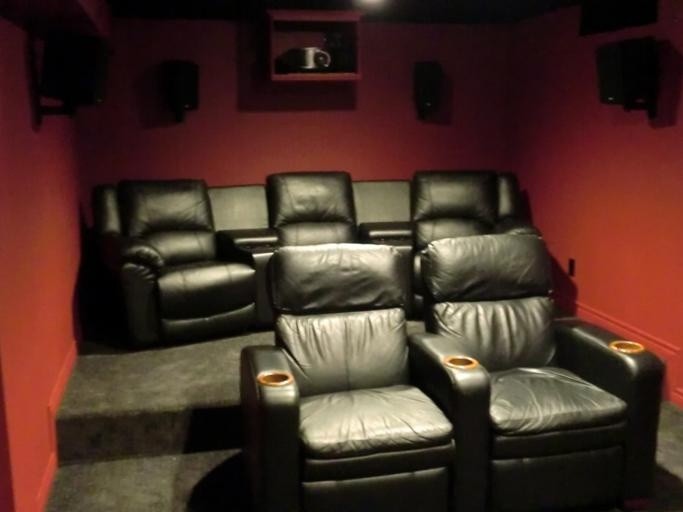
[407,168,519,312]
[99,178,253,341]
[237,243,456,510]
[264,168,360,245]
[412,229,663,511]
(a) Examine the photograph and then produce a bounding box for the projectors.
[276,41,355,72]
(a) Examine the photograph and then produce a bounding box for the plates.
[298,64,328,70]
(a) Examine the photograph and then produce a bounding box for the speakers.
[415,61,442,111]
[597,35,656,105]
[41,26,112,105]
[159,59,199,111]
[581,0,657,36]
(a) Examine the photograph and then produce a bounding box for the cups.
[300,47,330,67]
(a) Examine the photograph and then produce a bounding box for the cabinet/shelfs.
[267,8,362,82]
